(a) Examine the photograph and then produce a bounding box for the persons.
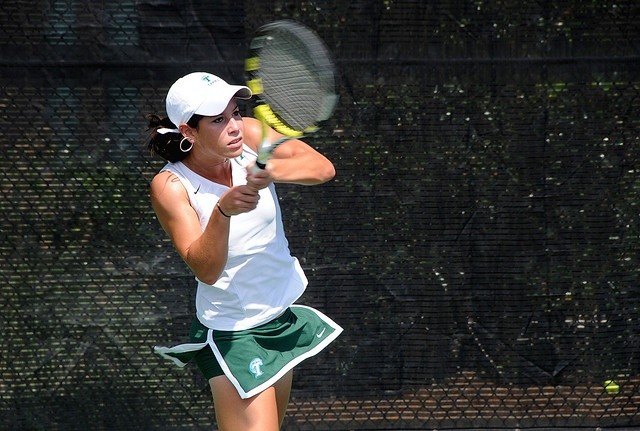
[148,71,336,431]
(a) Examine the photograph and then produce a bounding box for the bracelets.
[216,202,230,218]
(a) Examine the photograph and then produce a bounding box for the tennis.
[604,379,619,395]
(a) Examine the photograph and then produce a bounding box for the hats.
[166,72,252,130]
